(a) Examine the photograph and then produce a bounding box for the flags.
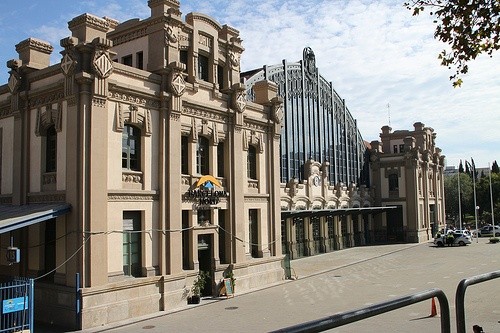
[459,159,472,177]
[491,160,499,175]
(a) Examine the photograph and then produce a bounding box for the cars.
[451,230,473,239]
[474,224,500,237]
[433,233,471,247]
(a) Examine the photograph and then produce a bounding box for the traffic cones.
[430,296,437,315]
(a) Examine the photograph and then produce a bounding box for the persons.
[447,232,451,246]
[471,159,477,181]
[449,233,455,247]
